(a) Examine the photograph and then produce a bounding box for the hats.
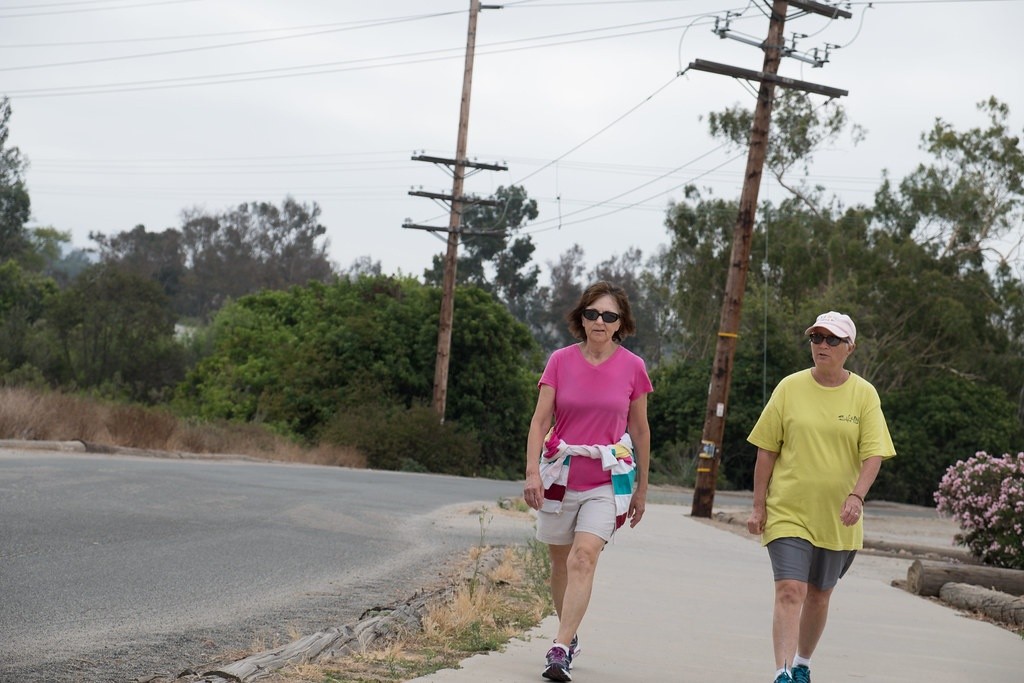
[804,311,856,346]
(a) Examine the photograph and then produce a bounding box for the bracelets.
[849,493,864,505]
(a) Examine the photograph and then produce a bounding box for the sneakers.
[542,646,571,680]
[774,666,792,683]
[553,633,581,662]
[791,665,810,683]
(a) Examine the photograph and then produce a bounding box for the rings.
[855,513,858,515]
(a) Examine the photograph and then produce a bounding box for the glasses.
[810,333,844,346]
[582,309,621,323]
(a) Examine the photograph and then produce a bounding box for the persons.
[524,281,654,681]
[747,311,896,683]
